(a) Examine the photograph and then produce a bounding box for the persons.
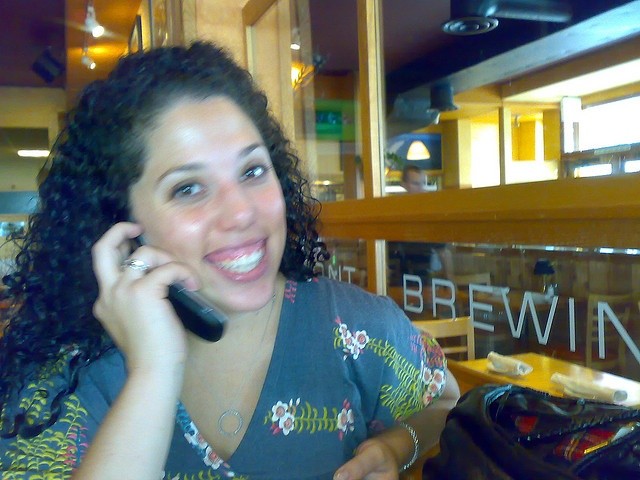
[387,166,453,285]
[1,42,460,480]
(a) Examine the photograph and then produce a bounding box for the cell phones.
[119,226,228,343]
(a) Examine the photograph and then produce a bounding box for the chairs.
[446,273,513,355]
[587,292,633,370]
[406,314,476,366]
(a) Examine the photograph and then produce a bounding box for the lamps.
[533,260,554,293]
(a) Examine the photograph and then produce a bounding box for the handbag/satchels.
[422,383,640,480]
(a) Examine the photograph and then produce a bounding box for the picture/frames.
[128,14,143,55]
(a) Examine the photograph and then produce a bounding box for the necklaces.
[190,291,278,440]
